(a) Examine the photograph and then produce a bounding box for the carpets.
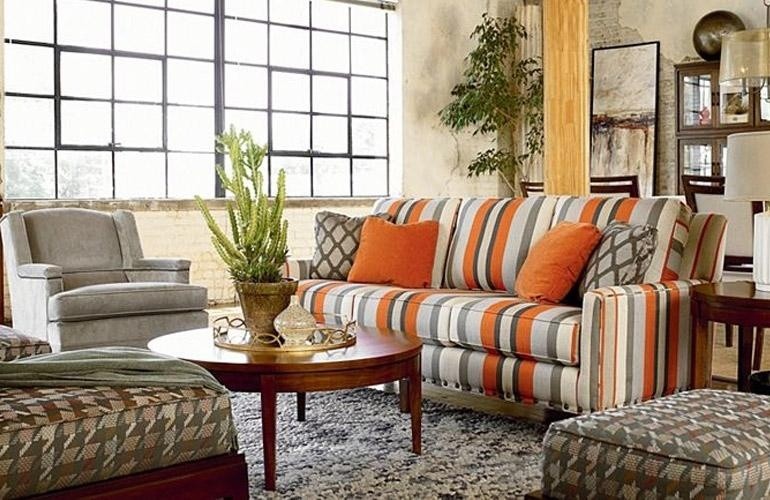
[229,388,550,500]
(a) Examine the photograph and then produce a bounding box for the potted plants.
[194,121,300,340]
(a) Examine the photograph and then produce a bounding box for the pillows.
[513,220,657,306]
[309,209,440,290]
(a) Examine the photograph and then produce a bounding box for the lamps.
[725,130,769,290]
[717,0,770,102]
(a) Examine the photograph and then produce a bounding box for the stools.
[524,386,770,498]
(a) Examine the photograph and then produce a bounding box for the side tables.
[688,282,768,393]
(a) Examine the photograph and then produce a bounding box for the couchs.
[278,195,728,425]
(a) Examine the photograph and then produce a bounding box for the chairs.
[0,207,209,352]
[517,173,762,276]
